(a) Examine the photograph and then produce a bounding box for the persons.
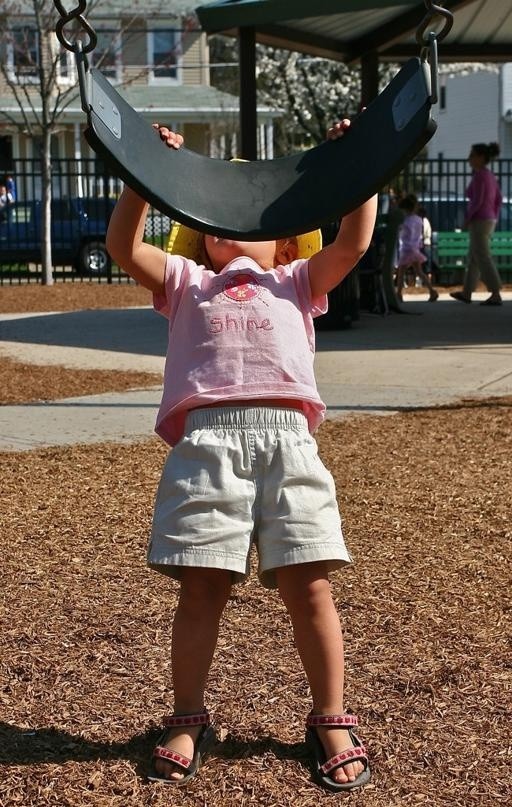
[6,176,16,221]
[450,142,503,305]
[104,123,378,789]
[407,207,433,286]
[396,194,438,303]
[1,186,14,222]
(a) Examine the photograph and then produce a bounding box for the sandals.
[306,708,372,789]
[145,704,215,784]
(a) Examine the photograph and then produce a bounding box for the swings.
[54,1,454,243]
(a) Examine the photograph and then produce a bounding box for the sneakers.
[427,290,504,305]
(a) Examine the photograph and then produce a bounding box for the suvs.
[1,196,146,280]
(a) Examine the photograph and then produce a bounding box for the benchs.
[431,230,512,289]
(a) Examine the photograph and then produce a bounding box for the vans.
[378,194,512,290]
